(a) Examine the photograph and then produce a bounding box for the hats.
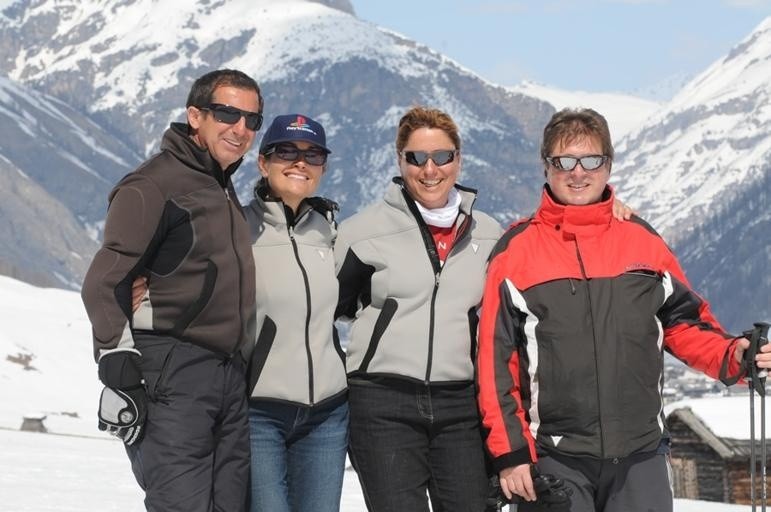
[259,113,332,155]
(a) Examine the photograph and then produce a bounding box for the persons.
[128,111,353,512]
[80,67,266,512]
[333,106,643,512]
[473,107,771,512]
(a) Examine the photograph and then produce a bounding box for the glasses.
[398,149,457,167]
[544,154,609,171]
[263,143,328,167]
[199,103,264,131]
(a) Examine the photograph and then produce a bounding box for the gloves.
[97,349,149,449]
[485,463,575,510]
